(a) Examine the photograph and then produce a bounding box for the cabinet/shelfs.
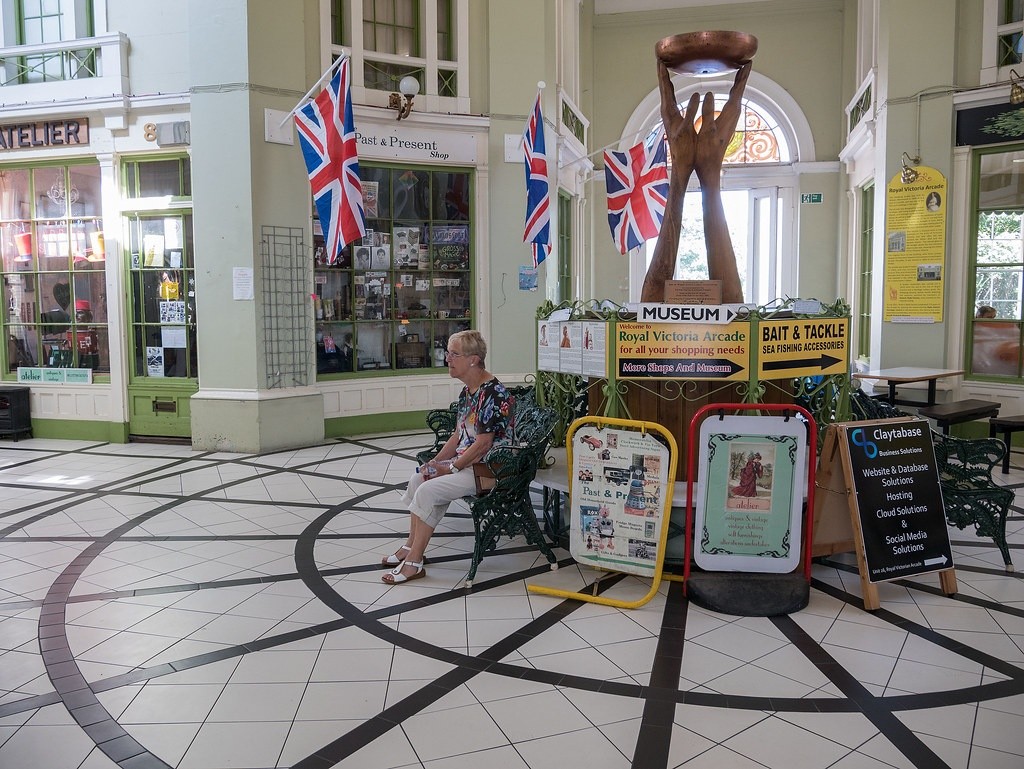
[0,385,34,442]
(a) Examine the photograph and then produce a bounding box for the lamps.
[389,76,419,120]
[901,151,922,185]
[1009,68,1024,103]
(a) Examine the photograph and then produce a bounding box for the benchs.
[796,379,1016,575]
[989,414,1023,476]
[416,380,560,588]
[915,398,1001,440]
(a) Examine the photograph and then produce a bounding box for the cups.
[14,233,32,260]
[438,311,449,319]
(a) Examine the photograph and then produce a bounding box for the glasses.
[445,350,482,360]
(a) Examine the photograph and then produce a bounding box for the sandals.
[382,542,411,565]
[381,557,426,584]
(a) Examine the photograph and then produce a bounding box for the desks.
[849,368,965,408]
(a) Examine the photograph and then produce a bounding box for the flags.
[292,56,366,263]
[522,92,552,267]
[605,123,685,254]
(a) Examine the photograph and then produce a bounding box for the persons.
[977,305,997,318]
[373,248,388,268]
[76,309,93,329]
[344,332,362,371]
[356,248,369,268]
[579,469,593,481]
[381,330,520,585]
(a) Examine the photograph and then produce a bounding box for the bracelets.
[450,463,459,474]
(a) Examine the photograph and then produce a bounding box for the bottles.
[416,460,455,476]
[336,291,345,320]
[145,246,155,265]
